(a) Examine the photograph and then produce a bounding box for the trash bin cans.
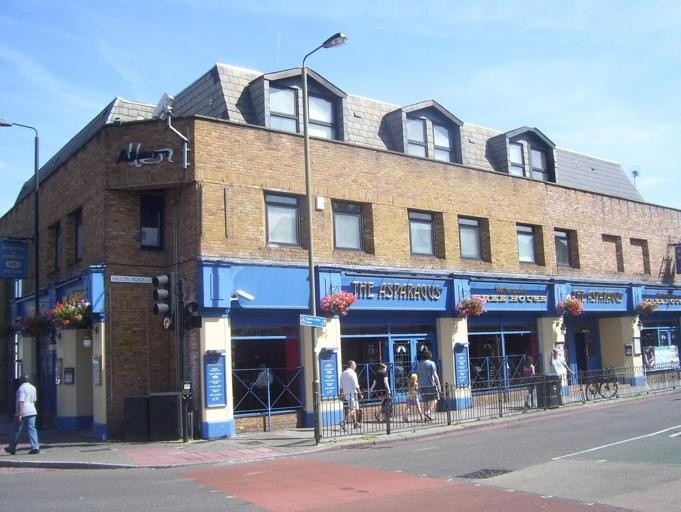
[536,374,561,408]
[122,392,180,442]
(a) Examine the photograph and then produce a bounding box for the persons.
[522,357,536,409]
[255,363,274,410]
[338,360,364,432]
[644,349,655,370]
[416,351,441,420]
[370,363,393,423]
[546,348,574,406]
[4,373,41,455]
[402,374,426,422]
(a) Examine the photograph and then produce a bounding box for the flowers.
[456,294,489,319]
[637,299,659,317]
[319,289,357,317]
[18,289,93,339]
[559,294,585,319]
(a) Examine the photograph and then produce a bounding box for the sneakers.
[4,446,15,455]
[28,448,40,454]
[339,419,362,431]
[401,410,435,422]
[374,411,381,421]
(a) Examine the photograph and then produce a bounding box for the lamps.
[152,94,190,143]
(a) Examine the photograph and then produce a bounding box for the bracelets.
[358,393,362,395]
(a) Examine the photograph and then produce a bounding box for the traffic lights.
[152,271,175,320]
[185,300,203,330]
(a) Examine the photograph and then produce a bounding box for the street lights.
[301,32,349,443]
[0,120,43,430]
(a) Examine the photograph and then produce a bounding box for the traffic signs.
[300,315,325,328]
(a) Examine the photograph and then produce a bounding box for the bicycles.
[587,367,618,400]
